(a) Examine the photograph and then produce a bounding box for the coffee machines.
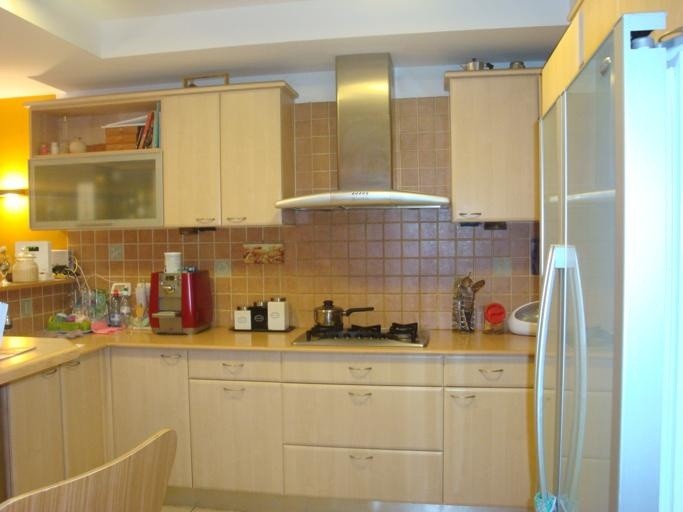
[147,269,213,335]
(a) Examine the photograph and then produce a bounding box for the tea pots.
[457,57,493,72]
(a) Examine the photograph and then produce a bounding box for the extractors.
[272,52,451,213]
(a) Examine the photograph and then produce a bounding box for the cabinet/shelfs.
[159,77,301,229]
[443,351,556,507]
[1,346,107,500]
[20,89,159,232]
[439,67,541,225]
[187,346,281,496]
[107,346,191,489]
[281,349,442,506]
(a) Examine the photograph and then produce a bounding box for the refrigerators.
[530,24,620,510]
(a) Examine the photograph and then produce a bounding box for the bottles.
[66,136,87,153]
[126,189,146,218]
[11,256,38,283]
[118,288,130,319]
[49,142,58,154]
[38,145,47,155]
[108,289,120,327]
[231,298,288,332]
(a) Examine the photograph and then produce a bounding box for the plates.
[44,329,92,335]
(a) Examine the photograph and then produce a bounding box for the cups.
[71,288,94,321]
[449,285,474,335]
[134,281,151,307]
[509,61,525,69]
[164,251,181,273]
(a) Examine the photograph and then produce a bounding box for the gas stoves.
[291,321,430,348]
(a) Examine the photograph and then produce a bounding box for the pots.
[312,298,374,326]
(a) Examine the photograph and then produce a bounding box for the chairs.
[0,426,181,512]
